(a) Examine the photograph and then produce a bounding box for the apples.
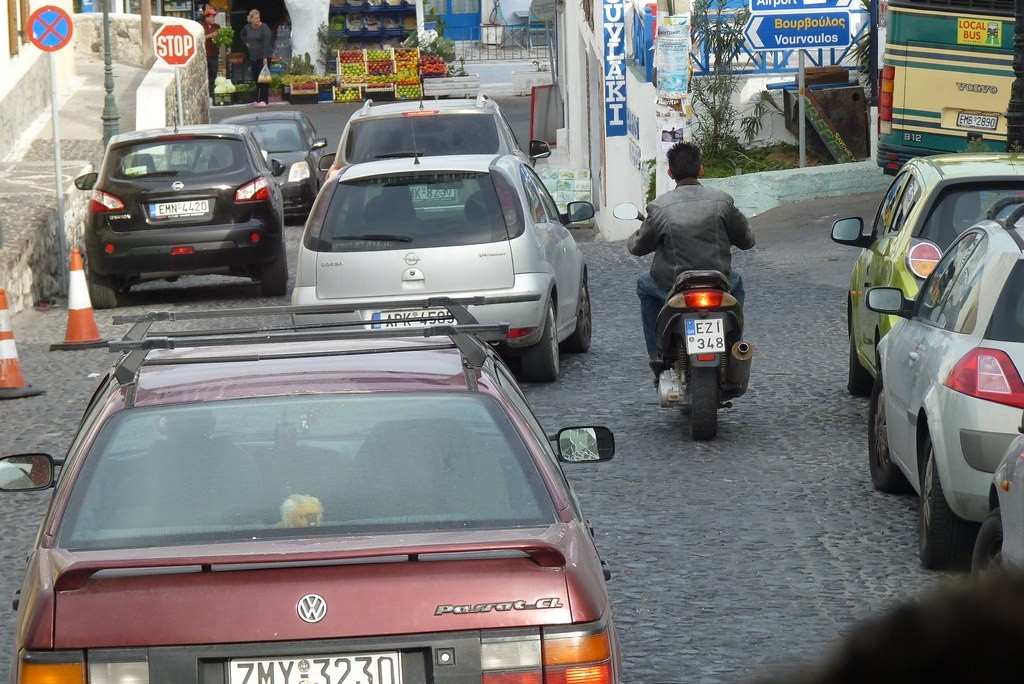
[335,49,420,102]
[421,54,445,74]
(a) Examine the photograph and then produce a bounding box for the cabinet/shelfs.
[326,0,415,47]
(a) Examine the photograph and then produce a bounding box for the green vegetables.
[234,73,281,98]
[212,28,235,47]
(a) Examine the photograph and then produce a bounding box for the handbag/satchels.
[257,65,272,84]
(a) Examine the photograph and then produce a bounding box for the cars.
[75,123,288,307]
[219,110,328,222]
[830,148,1024,397]
[970,409,1024,603]
[291,158,596,383]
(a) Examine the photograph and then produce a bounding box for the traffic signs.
[742,10,850,51]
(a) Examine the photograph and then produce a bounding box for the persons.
[628,141,755,379]
[240,9,273,108]
[199,8,221,106]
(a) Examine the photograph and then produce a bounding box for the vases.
[480,23,504,49]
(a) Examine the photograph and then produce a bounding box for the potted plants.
[424,56,480,99]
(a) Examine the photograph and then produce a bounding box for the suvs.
[866,188,1024,567]
[318,99,551,233]
[0,317,624,684]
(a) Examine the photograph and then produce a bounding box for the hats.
[204,9,218,15]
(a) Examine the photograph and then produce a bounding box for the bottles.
[273,26,292,63]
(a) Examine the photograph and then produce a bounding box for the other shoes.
[256,101,267,108]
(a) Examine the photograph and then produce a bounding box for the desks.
[514,11,532,48]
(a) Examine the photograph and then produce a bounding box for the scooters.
[614,201,752,440]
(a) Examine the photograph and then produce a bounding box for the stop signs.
[153,23,196,66]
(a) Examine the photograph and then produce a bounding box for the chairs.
[144,438,274,525]
[453,123,481,151]
[496,0,554,51]
[464,191,498,221]
[131,154,156,175]
[365,184,420,222]
[277,127,295,144]
[346,414,511,516]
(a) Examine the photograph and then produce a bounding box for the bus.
[876,0,1019,177]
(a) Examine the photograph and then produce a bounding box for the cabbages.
[214,77,236,103]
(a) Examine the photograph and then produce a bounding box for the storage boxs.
[214,47,423,103]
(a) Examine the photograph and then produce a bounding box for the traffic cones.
[50,247,114,351]
[0,289,45,399]
